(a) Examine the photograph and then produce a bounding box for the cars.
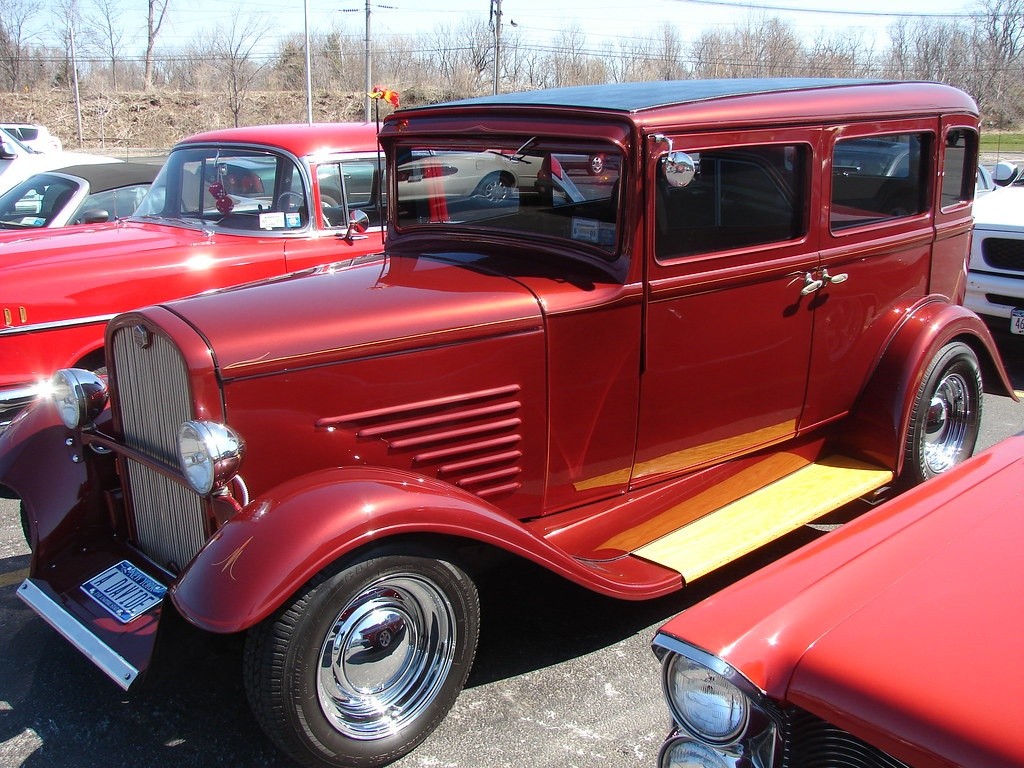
[1,78,1022,768]
[645,427,1024,768]
[787,137,1024,360]
[1,128,737,445]
